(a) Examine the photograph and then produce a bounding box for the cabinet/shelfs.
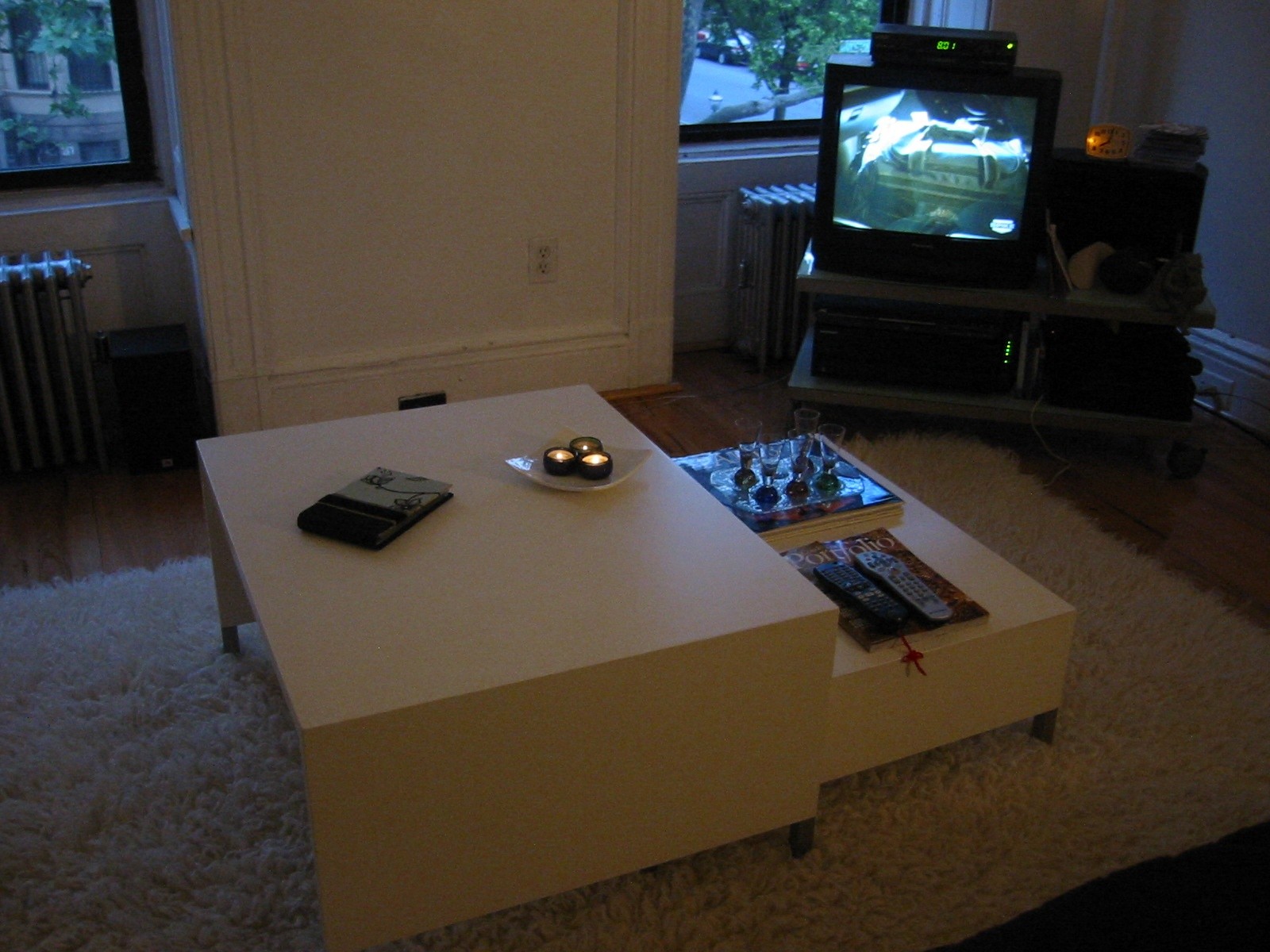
[788,239,1216,477]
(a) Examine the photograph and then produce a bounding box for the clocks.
[1086,124,1131,159]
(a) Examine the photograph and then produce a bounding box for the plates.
[505,426,654,494]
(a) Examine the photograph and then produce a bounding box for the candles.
[549,443,607,464]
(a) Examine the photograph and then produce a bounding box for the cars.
[769,37,822,81]
[693,23,760,65]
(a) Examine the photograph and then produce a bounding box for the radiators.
[0,249,111,474]
[726,183,816,358]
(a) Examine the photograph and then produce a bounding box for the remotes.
[813,563,920,627]
[855,552,954,622]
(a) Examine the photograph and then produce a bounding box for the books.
[296,467,454,552]
[672,436,905,541]
[778,527,990,654]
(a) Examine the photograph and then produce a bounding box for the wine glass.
[793,408,819,471]
[733,419,762,487]
[815,424,846,492]
[756,434,783,502]
[786,428,815,501]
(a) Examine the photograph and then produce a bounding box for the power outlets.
[1198,370,1233,406]
[529,239,558,283]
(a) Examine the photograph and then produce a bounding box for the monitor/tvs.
[819,51,1068,286]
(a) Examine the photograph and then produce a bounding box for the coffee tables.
[671,435,1074,783]
[195,385,837,952]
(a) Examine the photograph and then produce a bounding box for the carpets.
[1,435,1270,950]
